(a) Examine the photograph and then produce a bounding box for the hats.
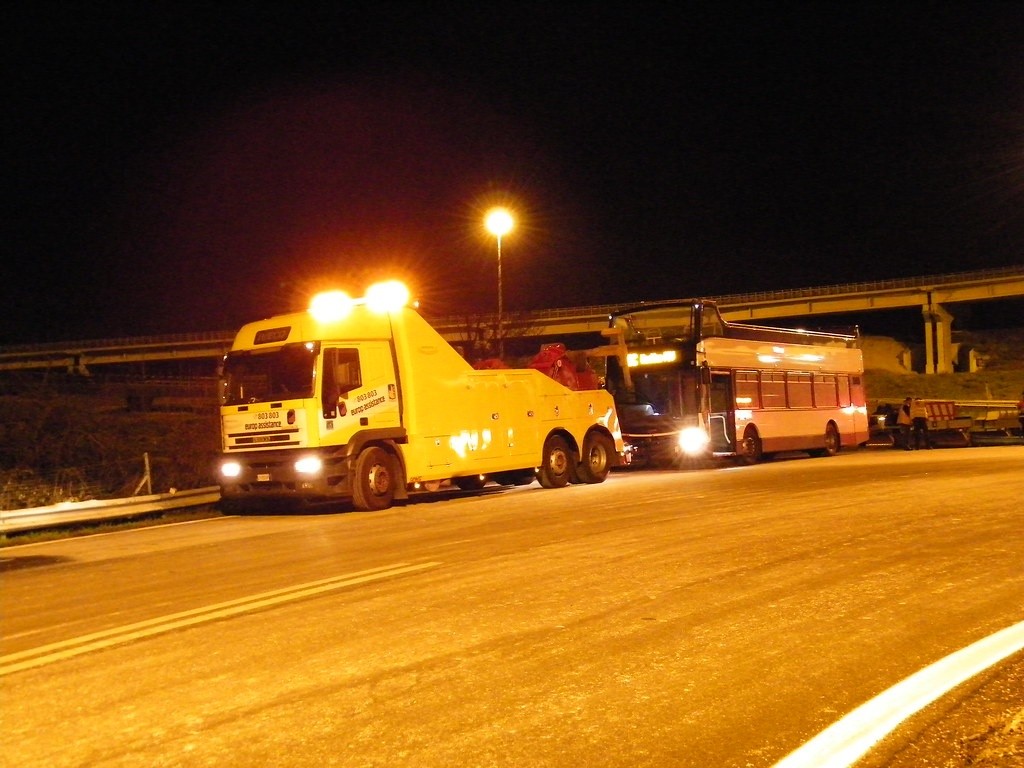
[904,397,912,402]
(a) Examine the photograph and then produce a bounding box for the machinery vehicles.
[583,298,869,466]
[219,305,630,512]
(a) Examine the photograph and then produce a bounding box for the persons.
[896,396,914,451]
[1016,391,1024,426]
[910,396,935,451]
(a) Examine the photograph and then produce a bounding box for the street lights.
[487,210,514,361]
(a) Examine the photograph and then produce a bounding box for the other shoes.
[915,448,919,449]
[928,446,932,449]
[906,447,913,450]
[904,448,907,450]
